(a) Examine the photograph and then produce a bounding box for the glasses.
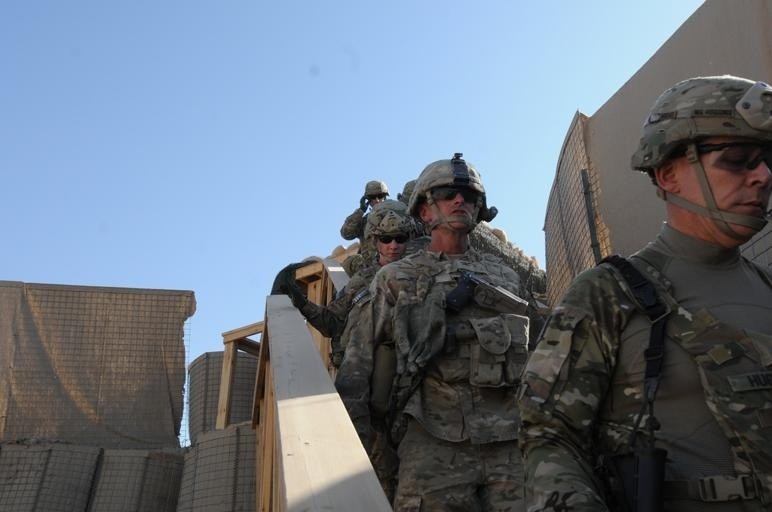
[367,193,385,200]
[709,143,770,173]
[380,234,406,243]
[432,187,476,201]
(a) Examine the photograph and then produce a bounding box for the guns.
[446,274,484,313]
[581,169,602,267]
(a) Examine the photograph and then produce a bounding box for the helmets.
[630,76,771,170]
[364,199,415,241]
[397,180,417,204]
[405,158,485,216]
[365,181,387,197]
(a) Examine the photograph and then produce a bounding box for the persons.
[276,178,432,500]
[515,75,772,512]
[341,152,552,510]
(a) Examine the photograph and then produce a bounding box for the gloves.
[279,271,309,309]
[360,196,371,212]
